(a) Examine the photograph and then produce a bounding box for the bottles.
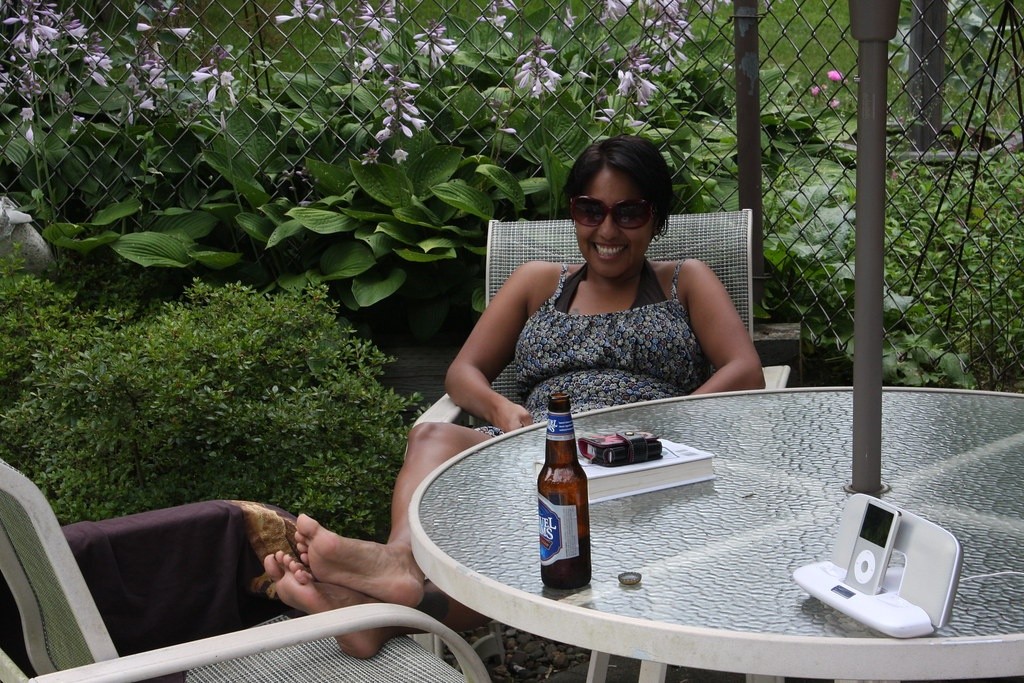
[537,392,592,590]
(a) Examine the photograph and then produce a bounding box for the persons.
[263,134,766,660]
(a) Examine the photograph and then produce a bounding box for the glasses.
[569,195,654,229]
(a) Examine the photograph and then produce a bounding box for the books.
[534,439,716,508]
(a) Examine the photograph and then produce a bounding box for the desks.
[406,387,1024,683]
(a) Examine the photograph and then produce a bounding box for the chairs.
[411,207,792,431]
[0,457,493,683]
